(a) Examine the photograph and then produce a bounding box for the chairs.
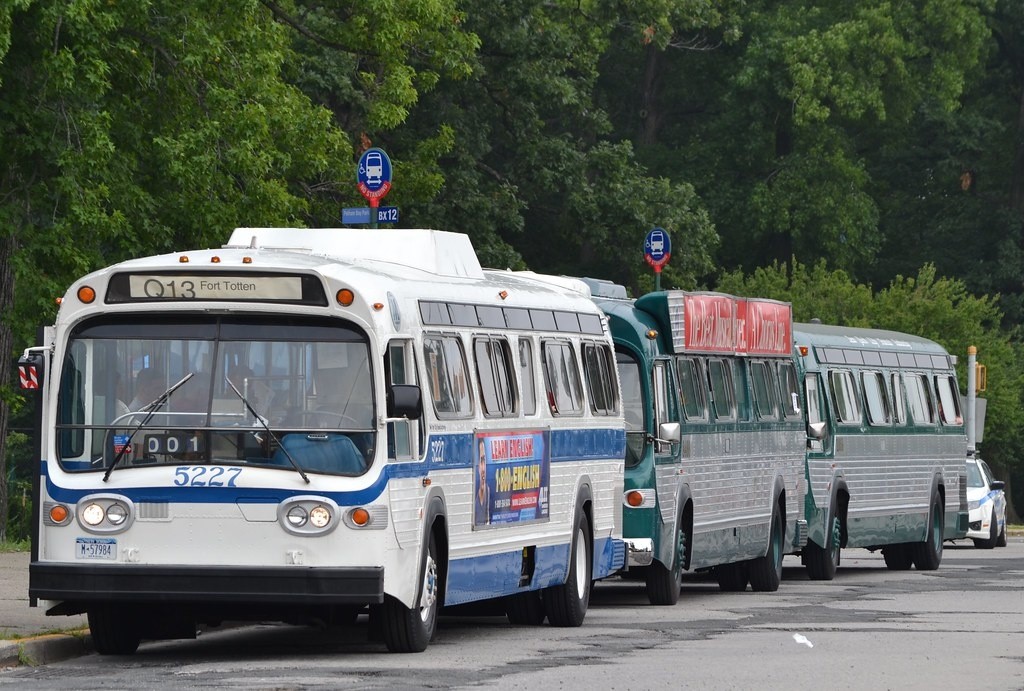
[313,403,374,476]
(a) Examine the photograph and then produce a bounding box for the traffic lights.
[976,366,987,392]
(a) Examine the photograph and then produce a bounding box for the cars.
[965,457,1007,548]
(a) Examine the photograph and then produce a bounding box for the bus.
[791,323,967,579]
[583,277,809,606]
[20,229,628,660]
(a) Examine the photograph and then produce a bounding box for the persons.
[128,368,189,428]
[92,370,153,455]
